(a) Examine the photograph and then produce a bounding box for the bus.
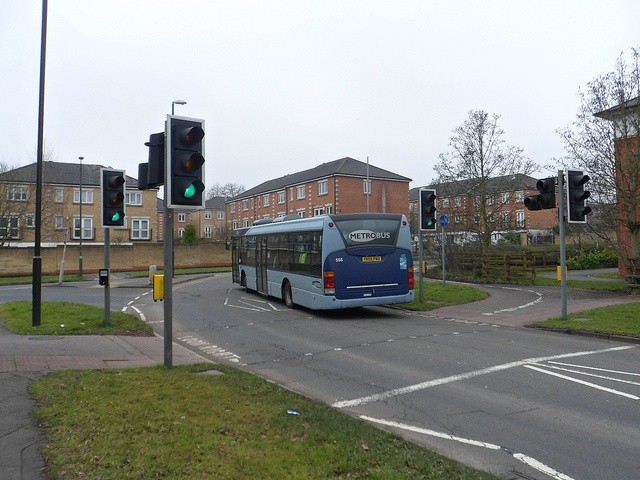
[226,213,414,310]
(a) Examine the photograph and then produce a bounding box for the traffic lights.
[565,168,591,223]
[524,177,556,211]
[100,167,127,228]
[167,114,206,211]
[420,188,438,233]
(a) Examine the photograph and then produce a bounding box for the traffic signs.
[439,214,448,228]
